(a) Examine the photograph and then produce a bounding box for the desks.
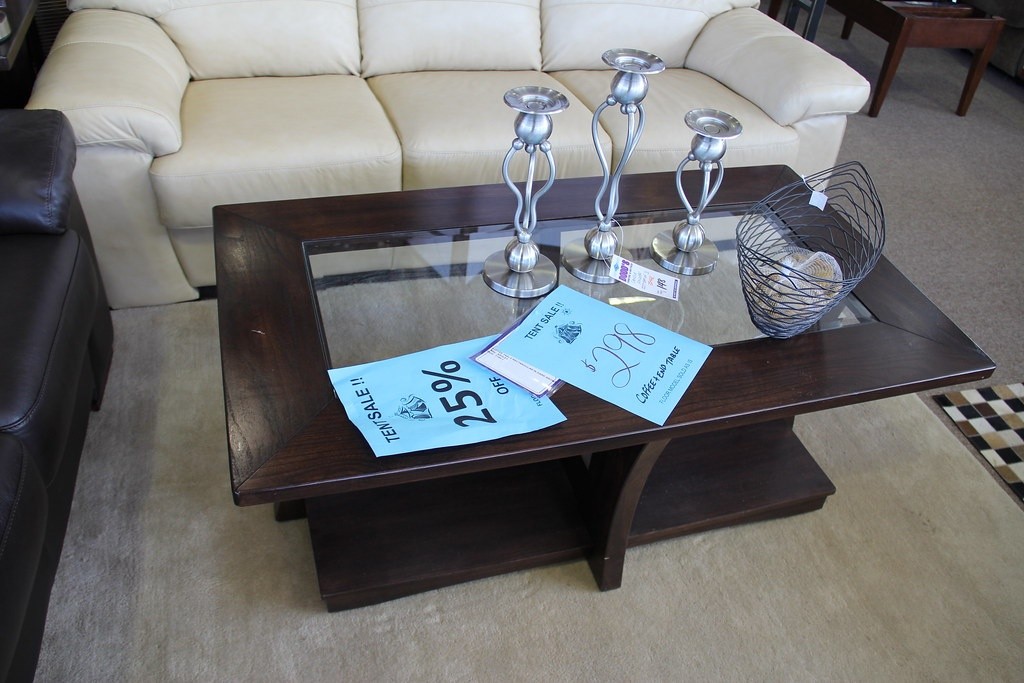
[767,0,1006,117]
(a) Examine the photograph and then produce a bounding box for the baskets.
[735,160,885,341]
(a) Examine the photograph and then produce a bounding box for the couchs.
[30,0,872,309]
[0,110,114,683]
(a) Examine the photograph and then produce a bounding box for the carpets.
[932,382,1024,505]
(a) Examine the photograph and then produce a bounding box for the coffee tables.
[212,164,998,612]
